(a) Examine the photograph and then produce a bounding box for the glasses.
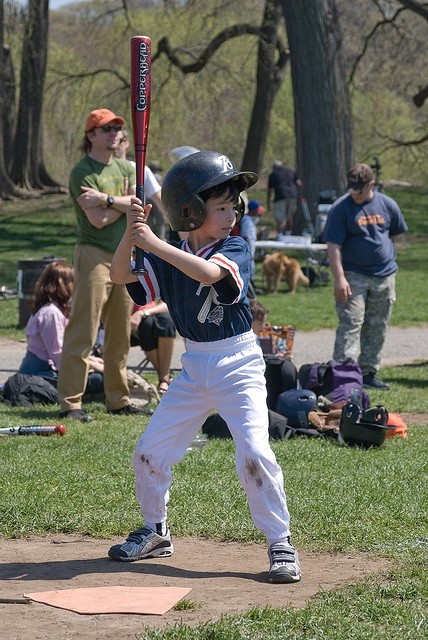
[344,183,363,196]
[95,125,122,132]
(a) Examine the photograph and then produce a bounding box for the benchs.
[255,240,329,285]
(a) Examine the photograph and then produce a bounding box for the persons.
[251,300,269,336]
[322,161,409,394]
[57,108,155,423]
[111,129,167,224]
[267,160,305,238]
[241,199,267,305]
[127,299,179,395]
[107,150,301,583]
[18,261,108,404]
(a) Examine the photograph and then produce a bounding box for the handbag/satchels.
[1,372,59,407]
[298,357,369,412]
[201,409,297,442]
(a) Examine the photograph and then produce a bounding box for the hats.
[160,150,259,231]
[376,412,406,439]
[339,402,399,450]
[85,108,126,132]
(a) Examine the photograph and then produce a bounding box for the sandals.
[157,380,170,395]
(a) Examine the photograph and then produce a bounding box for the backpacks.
[265,356,297,412]
[126,370,161,415]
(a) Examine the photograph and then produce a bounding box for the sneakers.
[363,373,390,390]
[267,537,301,583]
[107,526,174,561]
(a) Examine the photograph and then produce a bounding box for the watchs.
[105,192,113,209]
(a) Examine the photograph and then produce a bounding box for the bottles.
[347,388,362,421]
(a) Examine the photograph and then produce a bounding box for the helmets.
[275,389,317,431]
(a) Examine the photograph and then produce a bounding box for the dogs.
[261,249,311,294]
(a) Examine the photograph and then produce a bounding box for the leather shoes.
[107,405,154,417]
[59,410,92,423]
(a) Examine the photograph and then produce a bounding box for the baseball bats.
[127,35,154,277]
[298,194,316,235]
[0,424,69,437]
